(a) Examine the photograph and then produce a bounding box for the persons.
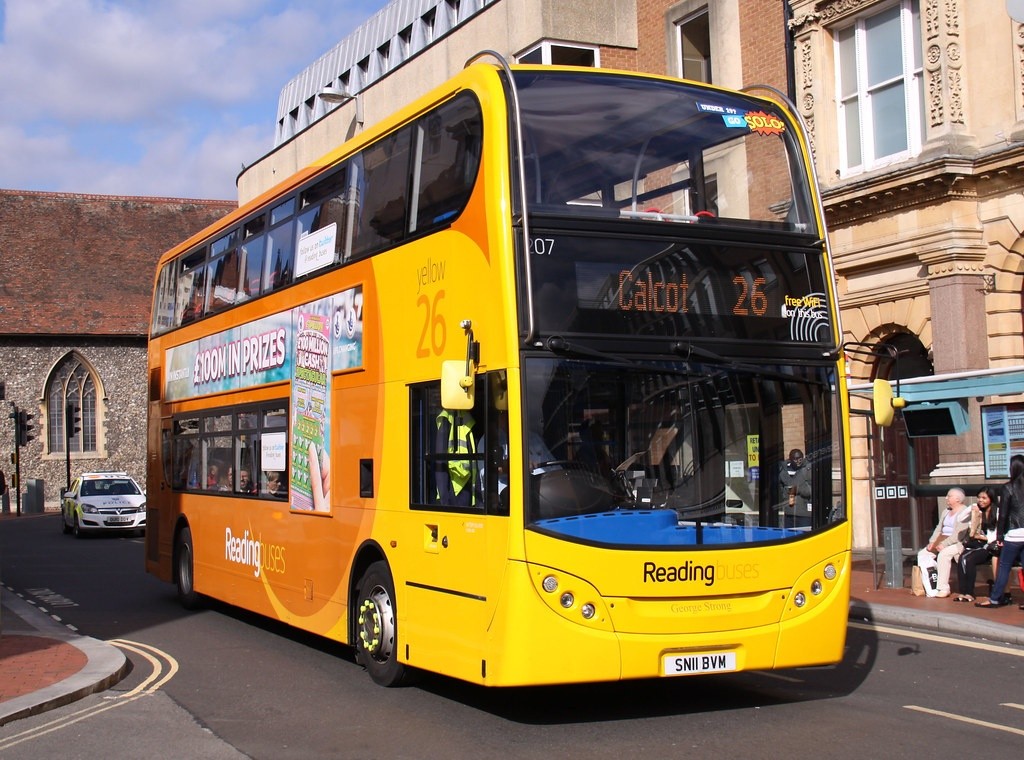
[264,471,282,496]
[197,465,218,490]
[974,454,1024,609]
[918,487,972,598]
[779,449,812,528]
[953,485,999,601]
[215,464,232,493]
[240,465,258,494]
[477,412,564,510]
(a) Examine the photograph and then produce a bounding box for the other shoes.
[973,600,999,608]
[926,589,950,597]
[953,594,976,602]
[1018,605,1024,610]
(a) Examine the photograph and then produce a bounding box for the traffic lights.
[69,403,81,438]
[20,409,34,446]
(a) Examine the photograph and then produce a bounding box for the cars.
[61,472,146,539]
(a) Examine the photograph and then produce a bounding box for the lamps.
[318,86,364,123]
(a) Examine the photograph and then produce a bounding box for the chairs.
[109,485,129,494]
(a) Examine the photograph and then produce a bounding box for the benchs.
[933,561,1023,589]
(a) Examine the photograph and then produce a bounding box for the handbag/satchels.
[910,561,940,597]
[963,528,983,549]
[933,535,949,550]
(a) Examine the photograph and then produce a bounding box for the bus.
[143,51,905,688]
